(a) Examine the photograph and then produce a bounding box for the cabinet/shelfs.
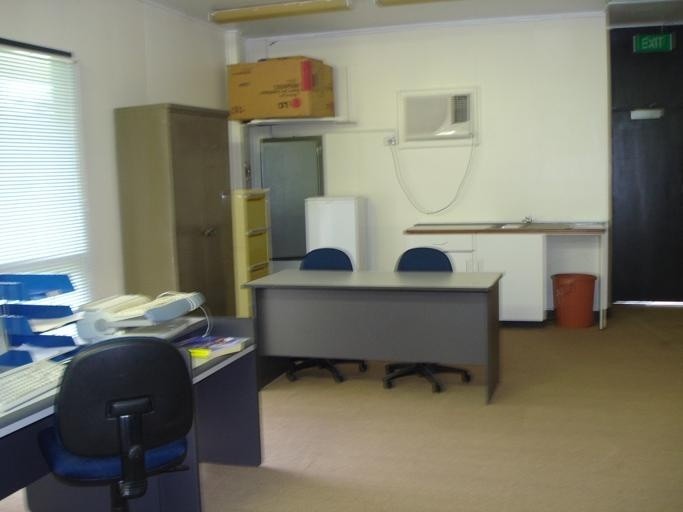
[404,222,609,331]
[113,102,237,316]
[232,188,270,318]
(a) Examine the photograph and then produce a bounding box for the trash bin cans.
[550,273,596,328]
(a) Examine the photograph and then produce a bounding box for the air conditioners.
[396,86,482,150]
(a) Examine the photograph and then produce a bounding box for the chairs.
[383,247,473,393]
[285,248,369,383]
[38,337,195,512]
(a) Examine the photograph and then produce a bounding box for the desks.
[0,318,262,501]
[246,268,503,404]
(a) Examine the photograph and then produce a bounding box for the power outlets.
[383,135,396,146]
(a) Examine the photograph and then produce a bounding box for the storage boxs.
[226,56,334,118]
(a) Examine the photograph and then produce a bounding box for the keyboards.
[1,359,66,413]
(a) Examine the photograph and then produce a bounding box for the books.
[179,335,250,355]
